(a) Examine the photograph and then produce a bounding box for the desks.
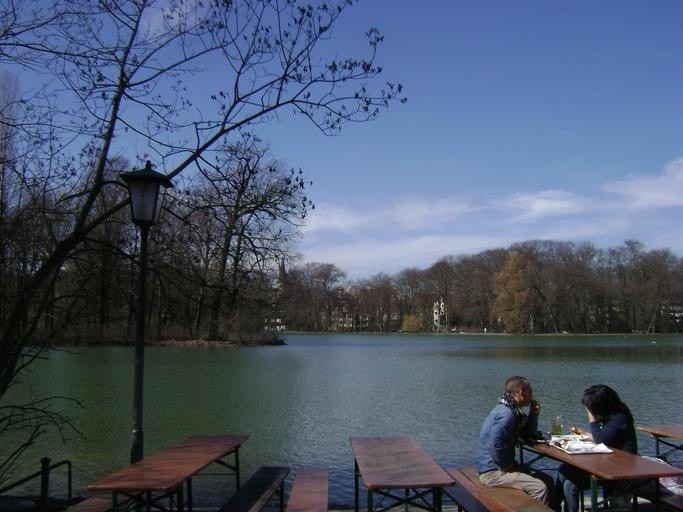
[518,421,682,509]
[348,433,456,511]
[86,434,249,510]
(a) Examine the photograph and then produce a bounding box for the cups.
[550,415,562,435]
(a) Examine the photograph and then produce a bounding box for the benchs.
[637,482,682,510]
[285,469,329,511]
[440,466,508,511]
[70,488,143,510]
[460,465,550,510]
[221,467,292,511]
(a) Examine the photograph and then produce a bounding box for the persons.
[476,374,558,510]
[556,385,637,512]
